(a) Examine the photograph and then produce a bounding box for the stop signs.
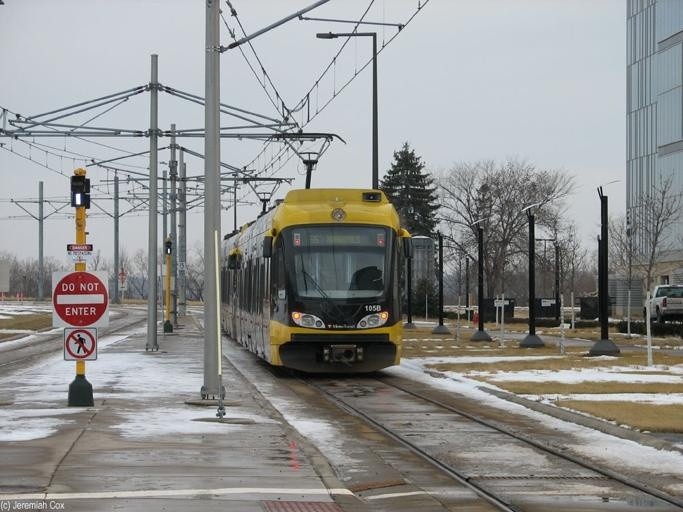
[52,271,109,327]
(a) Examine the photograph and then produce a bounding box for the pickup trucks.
[643,285,683,323]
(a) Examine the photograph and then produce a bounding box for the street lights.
[317,32,378,189]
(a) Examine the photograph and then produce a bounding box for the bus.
[221,189,413,373]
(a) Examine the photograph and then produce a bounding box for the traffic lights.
[69,176,90,208]
